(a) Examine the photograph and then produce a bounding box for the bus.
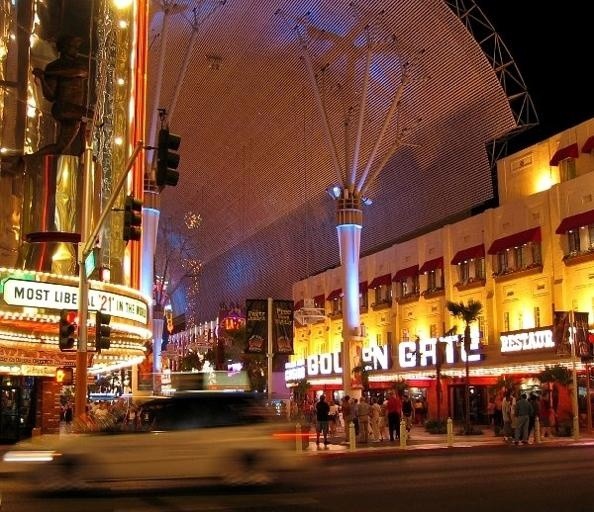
[171,371,251,392]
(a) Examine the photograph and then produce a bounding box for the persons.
[487,389,561,445]
[313,389,429,446]
[57,394,146,434]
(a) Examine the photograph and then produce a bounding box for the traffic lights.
[59,308,76,349]
[95,311,111,352]
[55,368,74,386]
[156,127,182,187]
[581,333,594,363]
[123,195,142,241]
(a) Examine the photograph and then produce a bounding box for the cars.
[0,393,315,497]
